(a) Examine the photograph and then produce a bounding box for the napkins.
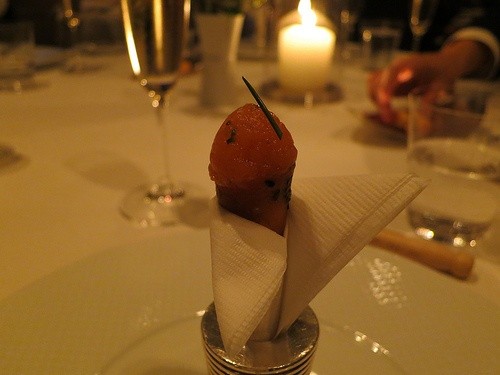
[206,170,429,358]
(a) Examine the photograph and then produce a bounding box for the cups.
[408,85,500,246]
[360,27,399,104]
[197,14,244,71]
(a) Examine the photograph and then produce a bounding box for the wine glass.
[119,0,208,222]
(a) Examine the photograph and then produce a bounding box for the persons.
[367,0,500,127]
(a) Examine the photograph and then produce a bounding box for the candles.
[275,0,336,95]
